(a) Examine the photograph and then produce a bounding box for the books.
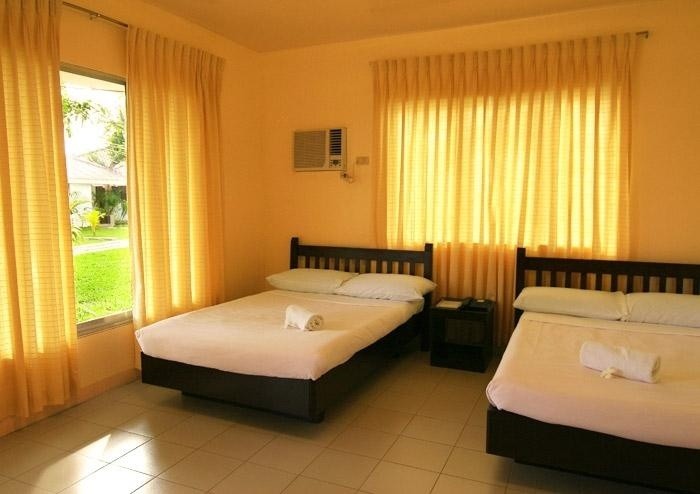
[436,298,462,309]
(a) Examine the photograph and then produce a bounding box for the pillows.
[265,263,436,303]
[511,277,699,331]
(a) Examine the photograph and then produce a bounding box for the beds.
[485,241,700,489]
[131,233,433,422]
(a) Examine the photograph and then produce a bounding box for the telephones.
[462,297,491,308]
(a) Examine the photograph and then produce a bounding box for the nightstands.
[428,297,495,373]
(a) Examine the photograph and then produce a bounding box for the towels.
[582,340,667,388]
[279,300,329,337]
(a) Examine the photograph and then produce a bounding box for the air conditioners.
[291,126,348,172]
